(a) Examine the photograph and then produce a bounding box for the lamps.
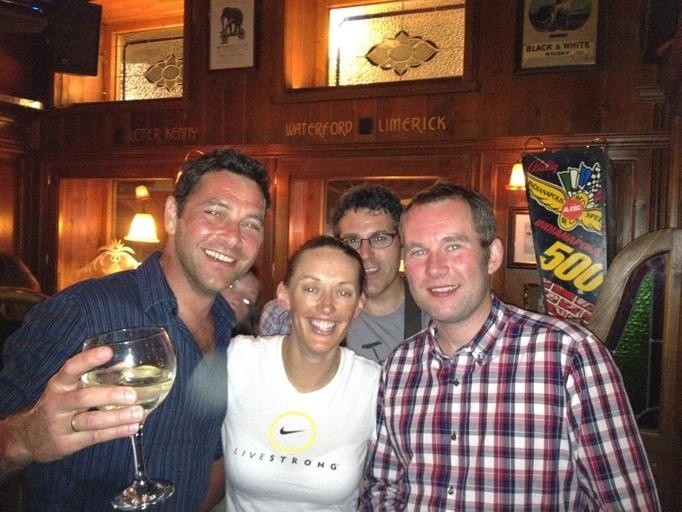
[122,182,161,247]
[504,134,547,192]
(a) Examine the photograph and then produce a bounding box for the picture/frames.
[203,0,262,78]
[510,0,609,79]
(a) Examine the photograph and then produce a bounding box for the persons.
[331,184,431,366]
[357,179,662,512]
[220,265,262,325]
[220,234,383,512]
[257,297,290,336]
[0,148,272,512]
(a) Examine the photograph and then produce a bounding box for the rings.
[71,410,84,432]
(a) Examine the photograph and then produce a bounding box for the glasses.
[337,233,396,250]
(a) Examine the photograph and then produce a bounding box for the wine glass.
[81,325,178,511]
